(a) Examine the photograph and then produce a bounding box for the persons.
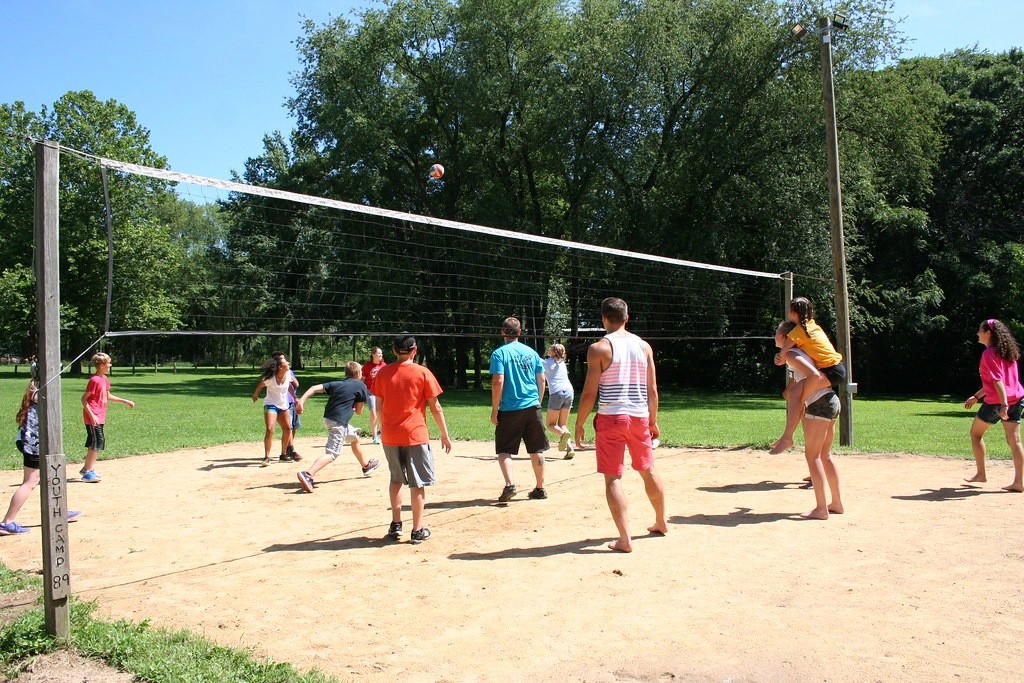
[539,343,576,459]
[574,296,670,552]
[294,360,382,491]
[488,317,549,502]
[768,295,846,520]
[369,329,452,542]
[360,344,387,445]
[0,360,84,536]
[79,351,135,483]
[251,349,302,466]
[962,320,1024,492]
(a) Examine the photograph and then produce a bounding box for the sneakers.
[298,471,314,494]
[499,484,517,501]
[388,521,403,537]
[362,458,381,476]
[81,470,101,483]
[262,457,271,466]
[412,526,431,542]
[288,445,304,461]
[528,487,547,499]
[68,510,82,520]
[279,454,293,461]
[78,467,101,478]
[0,521,31,535]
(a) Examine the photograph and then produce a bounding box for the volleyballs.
[429,164,444,178]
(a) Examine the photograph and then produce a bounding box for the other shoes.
[558,432,571,452]
[563,451,576,460]
[372,436,380,444]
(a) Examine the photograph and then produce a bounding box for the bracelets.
[974,395,979,400]
